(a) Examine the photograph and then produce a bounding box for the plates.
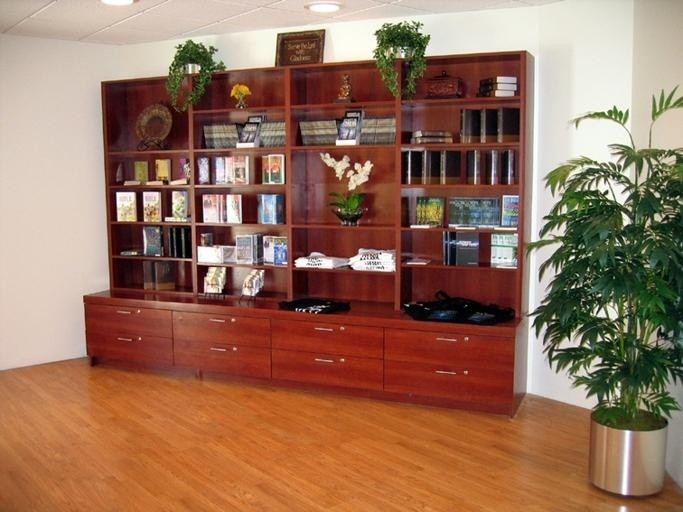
[134,103,173,145]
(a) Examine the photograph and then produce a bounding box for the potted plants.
[526,84,683,496]
[374,21,430,93]
[168,40,222,112]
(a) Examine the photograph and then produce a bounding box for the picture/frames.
[275,28,325,67]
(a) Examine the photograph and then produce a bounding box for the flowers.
[319,152,374,218]
[231,83,252,104]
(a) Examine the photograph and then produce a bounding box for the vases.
[235,95,249,108]
[332,204,369,226]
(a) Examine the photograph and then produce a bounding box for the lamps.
[100,0,139,6]
[303,3,342,14]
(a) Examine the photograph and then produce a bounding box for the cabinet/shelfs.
[83,49,535,418]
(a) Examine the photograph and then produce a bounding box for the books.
[407,75,521,270]
[114,153,287,298]
[203,109,397,148]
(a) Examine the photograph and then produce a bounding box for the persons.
[338,74,353,100]
